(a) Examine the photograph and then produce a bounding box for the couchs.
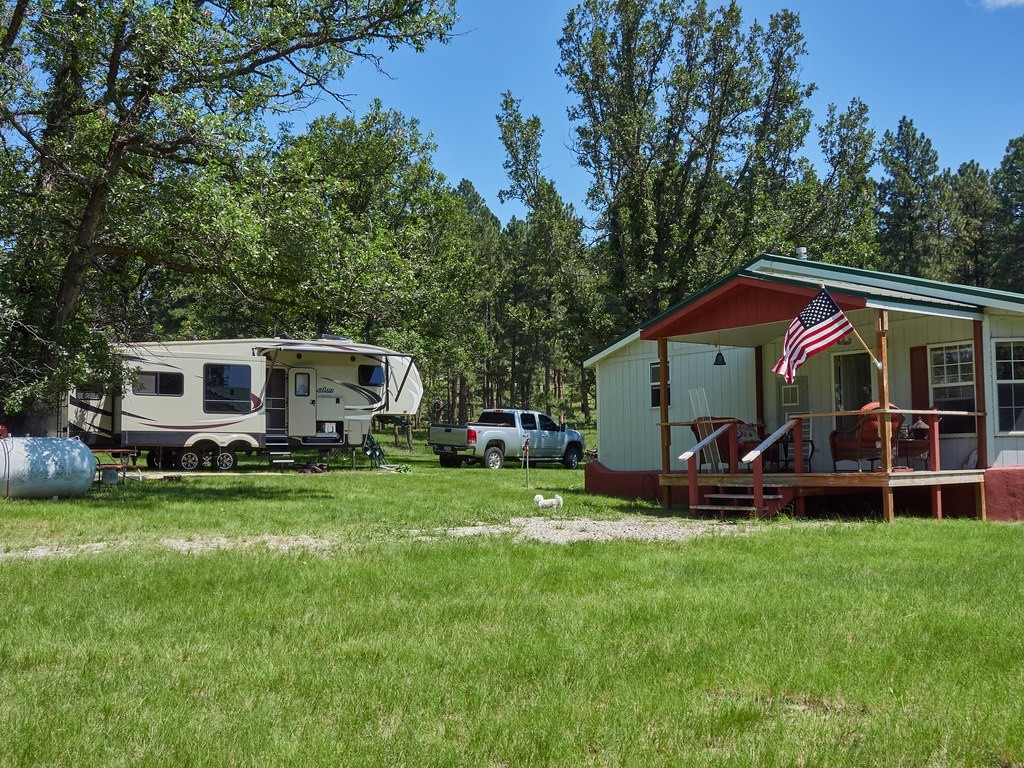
[689,415,766,471]
[828,401,906,474]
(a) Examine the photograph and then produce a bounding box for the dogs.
[534,494,563,511]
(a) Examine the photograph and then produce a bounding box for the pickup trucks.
[427,408,585,471]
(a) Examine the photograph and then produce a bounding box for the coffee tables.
[763,438,814,473]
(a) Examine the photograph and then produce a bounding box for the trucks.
[57,333,424,472]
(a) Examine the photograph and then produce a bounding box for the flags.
[771,287,854,385]
